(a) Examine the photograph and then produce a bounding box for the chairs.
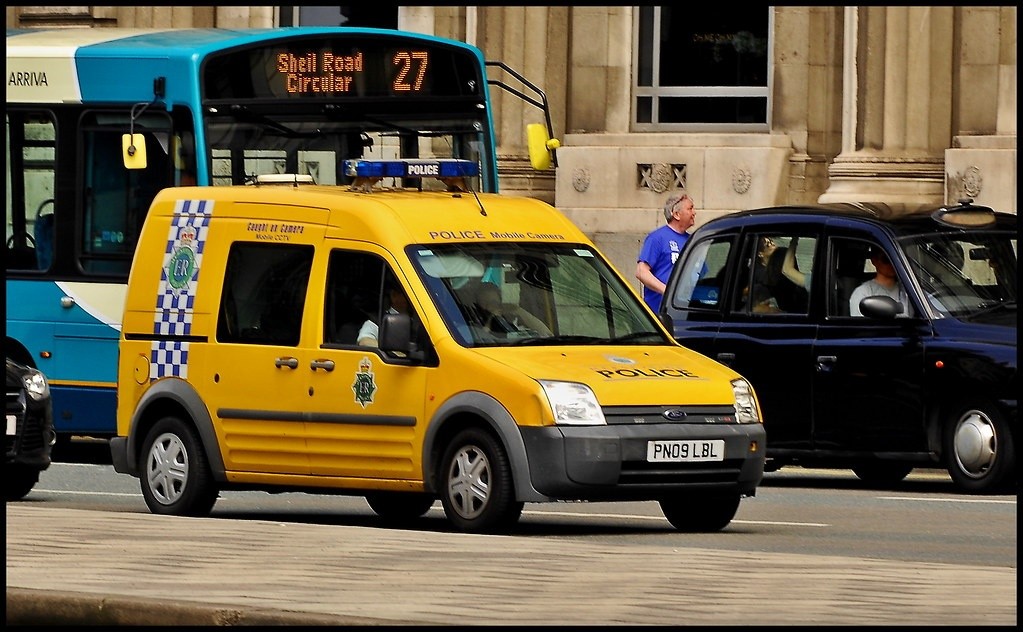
[5,199,55,271]
[767,246,809,314]
[924,240,986,318]
[452,261,499,321]
[832,240,871,316]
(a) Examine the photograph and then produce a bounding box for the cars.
[657,197,1020,494]
[3,356,56,501]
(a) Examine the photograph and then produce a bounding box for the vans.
[109,156,768,535]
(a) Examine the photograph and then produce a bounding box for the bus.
[5,25,559,453]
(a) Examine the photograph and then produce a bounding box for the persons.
[718,234,850,313]
[358,274,426,357]
[928,243,988,298]
[850,246,916,319]
[637,194,708,313]
[475,283,552,338]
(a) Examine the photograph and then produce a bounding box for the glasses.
[764,240,775,248]
[671,194,688,212]
[876,254,890,265]
